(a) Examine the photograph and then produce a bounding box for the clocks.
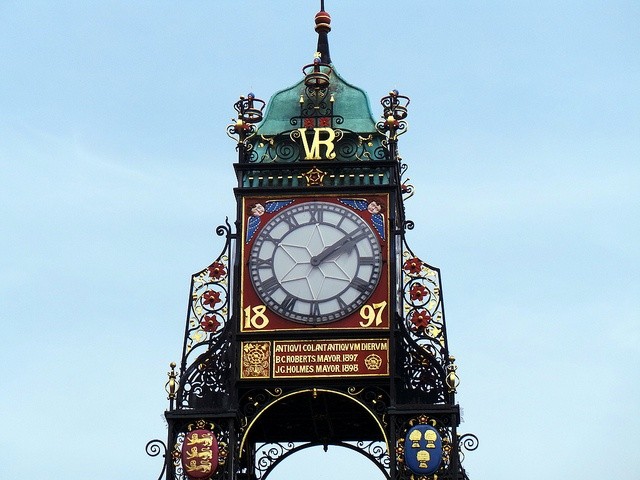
[242,195,390,332]
